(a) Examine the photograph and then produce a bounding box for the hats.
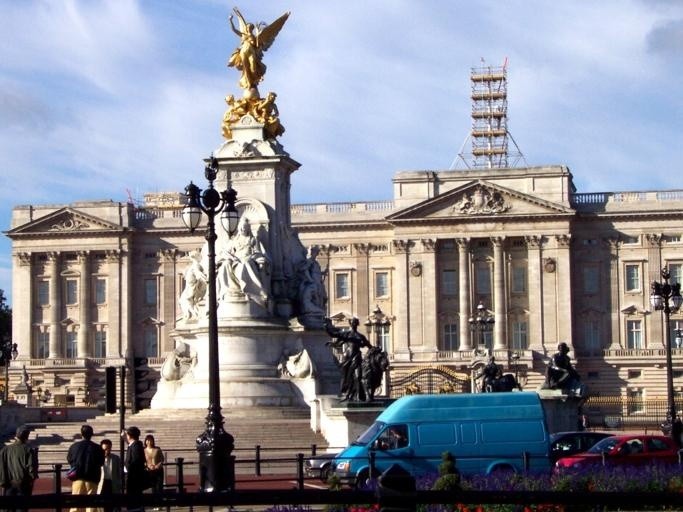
[16,425,29,436]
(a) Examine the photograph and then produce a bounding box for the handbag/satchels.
[66,467,77,480]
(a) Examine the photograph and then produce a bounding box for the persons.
[327,318,372,401]
[223,15,279,134]
[99,440,121,511]
[551,344,575,389]
[216,217,269,306]
[0,426,38,511]
[141,435,165,511]
[302,243,328,306]
[476,355,502,379]
[122,427,145,511]
[67,425,105,512]
[179,250,207,319]
[391,428,404,448]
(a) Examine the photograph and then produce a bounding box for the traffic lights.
[96,365,117,415]
[130,349,152,416]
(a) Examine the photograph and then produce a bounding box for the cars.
[543,427,614,463]
[304,452,336,484]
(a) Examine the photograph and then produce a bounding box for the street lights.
[638,309,652,349]
[466,303,495,343]
[511,350,520,381]
[0,341,19,404]
[648,264,682,434]
[150,271,166,358]
[363,305,396,347]
[363,318,372,345]
[673,326,683,349]
[178,146,245,495]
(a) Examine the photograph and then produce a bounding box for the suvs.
[549,433,681,488]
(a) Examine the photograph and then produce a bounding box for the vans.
[329,390,550,492]
